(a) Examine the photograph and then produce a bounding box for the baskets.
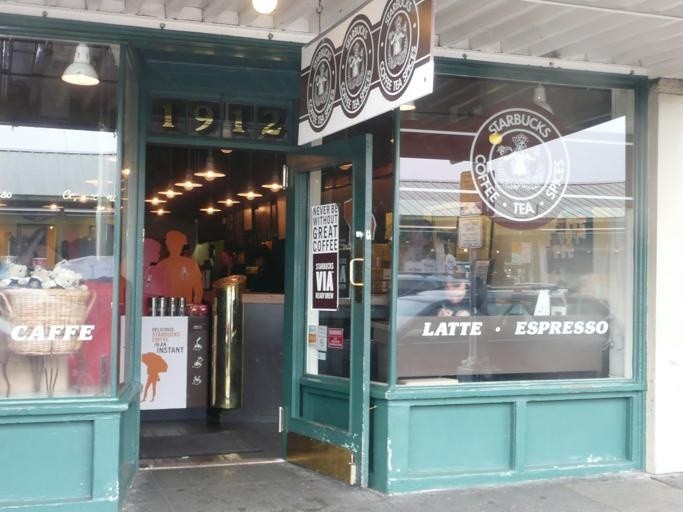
[0,287,96,356]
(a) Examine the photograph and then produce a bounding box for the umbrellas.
[142,352,168,373]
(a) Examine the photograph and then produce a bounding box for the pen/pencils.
[441,303,447,310]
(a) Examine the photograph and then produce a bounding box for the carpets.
[138,431,264,459]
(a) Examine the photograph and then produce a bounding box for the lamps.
[60,42,100,87]
[193,148,227,177]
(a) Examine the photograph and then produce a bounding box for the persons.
[142,239,171,316]
[157,230,204,305]
[428,274,478,317]
[140,360,160,402]
[408,220,438,262]
[218,250,239,278]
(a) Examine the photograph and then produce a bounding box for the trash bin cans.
[210,275,245,411]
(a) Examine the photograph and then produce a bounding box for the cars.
[397,273,611,383]
